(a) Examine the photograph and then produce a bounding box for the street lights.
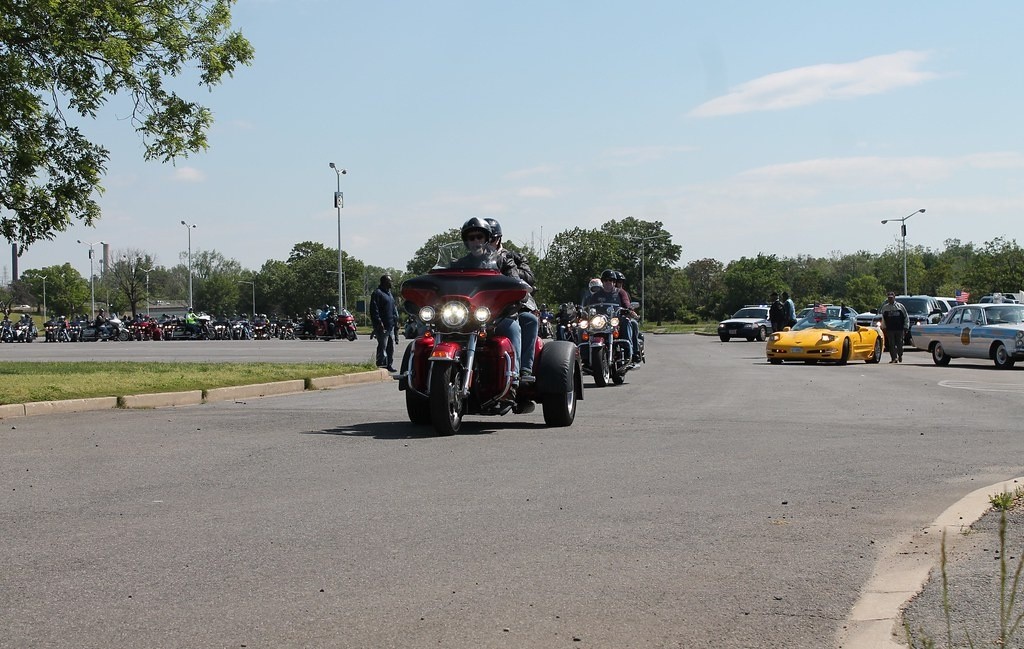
[613,235,673,327]
[881,210,925,296]
[139,220,256,315]
[36,240,104,320]
[329,163,347,314]
[327,271,347,309]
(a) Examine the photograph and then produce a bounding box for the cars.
[870,295,943,344]
[796,306,876,327]
[718,305,773,342]
[911,303,1024,370]
[934,297,972,322]
[978,293,1024,304]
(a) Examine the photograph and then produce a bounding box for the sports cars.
[766,316,884,365]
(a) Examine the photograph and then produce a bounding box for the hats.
[887,292,895,298]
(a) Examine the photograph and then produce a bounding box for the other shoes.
[510,384,518,398]
[635,353,640,362]
[889,357,900,364]
[520,369,532,384]
[386,365,397,372]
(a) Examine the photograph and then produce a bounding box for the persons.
[162,307,204,337]
[814,303,827,322]
[43,308,150,342]
[539,303,553,336]
[449,217,538,385]
[841,304,850,320]
[0,313,34,343]
[206,313,271,340]
[882,292,910,364]
[555,270,639,359]
[927,308,944,324]
[269,304,339,340]
[369,275,399,372]
[769,291,797,333]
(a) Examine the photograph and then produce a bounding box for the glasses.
[469,233,483,241]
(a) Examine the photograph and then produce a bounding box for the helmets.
[21,303,336,319]
[588,278,605,295]
[600,269,626,281]
[461,217,503,236]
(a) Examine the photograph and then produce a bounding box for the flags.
[956,289,971,303]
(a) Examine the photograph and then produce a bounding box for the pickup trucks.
[14,305,30,308]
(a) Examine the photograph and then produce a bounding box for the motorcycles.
[537,316,554,339]
[0,318,38,343]
[43,310,357,342]
[393,240,584,434]
[577,288,645,387]
[556,320,579,345]
